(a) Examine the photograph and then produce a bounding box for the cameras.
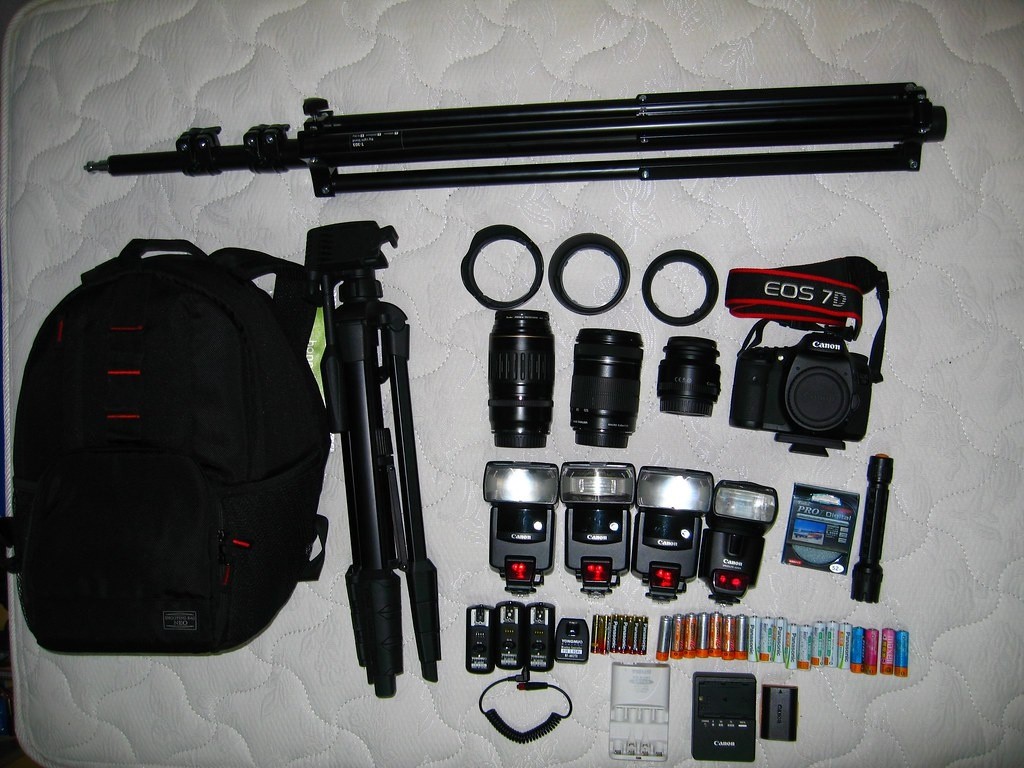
[729,335,873,457]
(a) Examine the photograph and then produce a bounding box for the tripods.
[84,80,950,202]
[303,220,441,698]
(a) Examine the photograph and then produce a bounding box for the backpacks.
[13,238,329,655]
